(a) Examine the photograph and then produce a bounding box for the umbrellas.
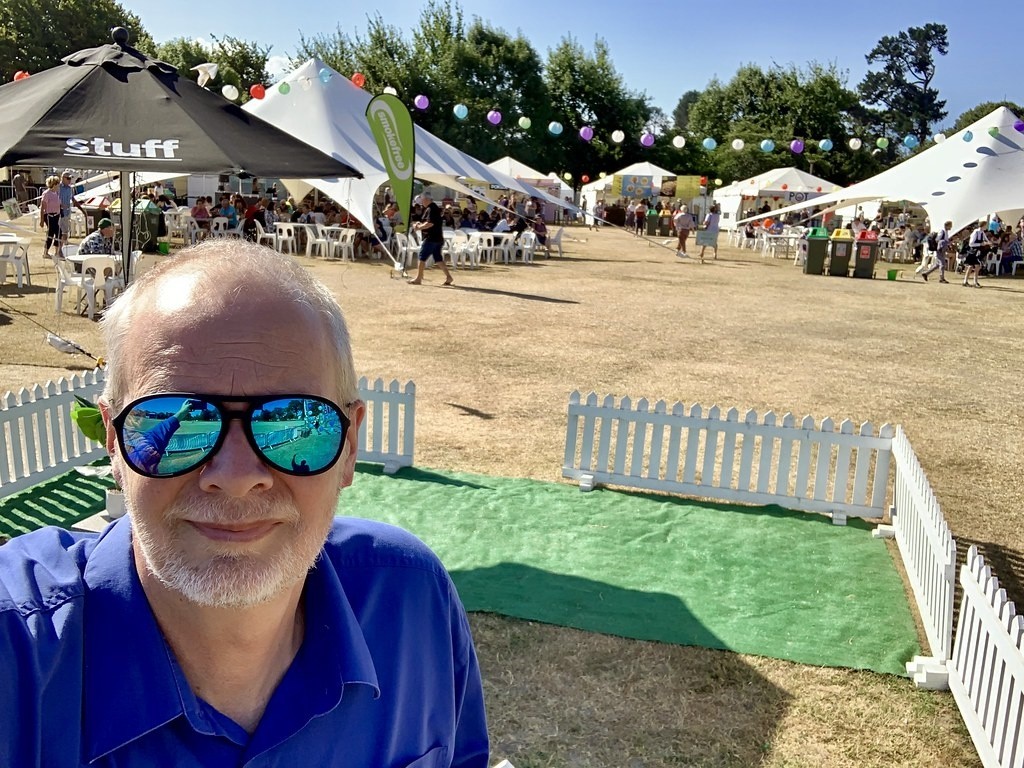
[0,25,363,283]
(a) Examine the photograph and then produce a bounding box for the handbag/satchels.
[964,251,980,265]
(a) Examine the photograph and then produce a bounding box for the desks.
[767,235,799,258]
[65,254,123,262]
[325,226,370,259]
[272,222,316,254]
[0,236,31,287]
[196,218,233,238]
[468,232,515,266]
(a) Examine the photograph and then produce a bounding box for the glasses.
[106,388,352,476]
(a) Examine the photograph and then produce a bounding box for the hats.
[98,218,119,230]
[62,172,74,179]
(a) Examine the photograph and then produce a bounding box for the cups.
[287,218,291,222]
[297,218,301,222]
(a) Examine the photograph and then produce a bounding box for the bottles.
[307,215,310,223]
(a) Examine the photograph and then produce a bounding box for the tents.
[580,160,706,227]
[733,105,1024,267]
[428,156,573,224]
[711,166,844,233]
[93,58,695,272]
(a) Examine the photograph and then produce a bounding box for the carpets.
[0,456,924,679]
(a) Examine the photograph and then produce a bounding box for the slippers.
[408,280,421,285]
[443,282,454,287]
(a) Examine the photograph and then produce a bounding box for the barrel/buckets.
[159,242,170,254]
[887,269,898,280]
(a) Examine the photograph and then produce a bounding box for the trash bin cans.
[830,229,855,276]
[646,210,658,236]
[854,231,878,278]
[659,210,672,236]
[800,228,829,275]
[108,198,122,250]
[131,199,162,253]
[672,210,683,236]
[80,196,111,236]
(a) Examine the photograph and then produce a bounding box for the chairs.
[877,237,1024,276]
[0,233,31,287]
[51,245,143,319]
[724,221,808,266]
[163,212,565,269]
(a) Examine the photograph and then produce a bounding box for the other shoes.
[44,254,52,259]
[962,283,972,287]
[939,279,949,283]
[922,273,928,282]
[974,283,982,288]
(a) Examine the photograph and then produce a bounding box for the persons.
[673,204,694,256]
[39,171,74,257]
[406,191,453,285]
[563,195,587,225]
[74,217,121,277]
[12,169,28,213]
[112,175,172,212]
[123,398,192,475]
[698,205,720,259]
[590,197,682,236]
[73,178,84,195]
[0,237,491,768]
[188,178,550,258]
[291,454,310,472]
[744,199,1024,288]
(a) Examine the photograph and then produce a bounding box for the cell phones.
[188,399,208,410]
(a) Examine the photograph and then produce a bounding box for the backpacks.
[928,230,945,251]
